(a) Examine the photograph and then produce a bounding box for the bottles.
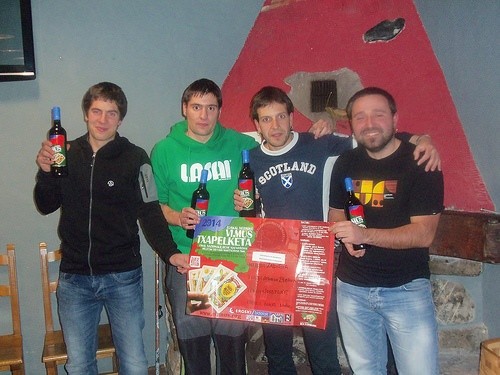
[186,170,210,239]
[238,150,256,217]
[49,107,68,177]
[344,177,371,251]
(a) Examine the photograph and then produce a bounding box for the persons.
[328,87,444,375]
[149,78,336,375]
[32,82,191,375]
[234,86,442,375]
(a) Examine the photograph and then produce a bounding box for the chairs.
[1,242,26,375]
[39,242,119,375]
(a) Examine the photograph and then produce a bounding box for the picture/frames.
[0,1,37,80]
